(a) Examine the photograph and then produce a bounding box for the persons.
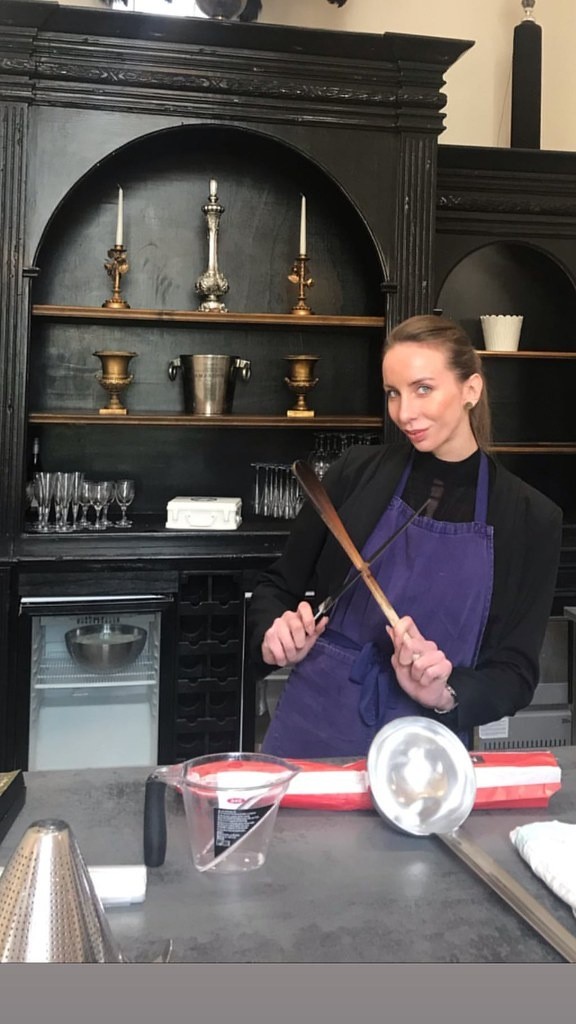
[241,314,563,758]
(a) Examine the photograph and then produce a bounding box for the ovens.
[19,592,172,771]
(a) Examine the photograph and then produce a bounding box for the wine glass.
[31,472,134,533]
[251,433,379,518]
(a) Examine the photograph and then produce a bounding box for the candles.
[116,183,123,245]
[209,178,217,196]
[299,192,307,256]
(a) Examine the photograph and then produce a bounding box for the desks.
[0,744,576,963]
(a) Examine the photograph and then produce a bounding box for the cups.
[169,355,251,415]
[480,314,523,351]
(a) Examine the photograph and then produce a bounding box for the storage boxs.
[165,496,243,531]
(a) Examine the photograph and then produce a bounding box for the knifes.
[312,498,432,621]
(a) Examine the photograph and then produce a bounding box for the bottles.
[512,0,542,150]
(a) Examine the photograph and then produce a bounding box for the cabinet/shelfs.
[0,0,576,772]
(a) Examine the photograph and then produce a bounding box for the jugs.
[144,752,301,872]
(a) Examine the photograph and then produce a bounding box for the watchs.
[434,684,460,714]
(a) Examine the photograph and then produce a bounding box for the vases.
[480,315,524,352]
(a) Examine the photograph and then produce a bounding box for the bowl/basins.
[64,623,147,675]
